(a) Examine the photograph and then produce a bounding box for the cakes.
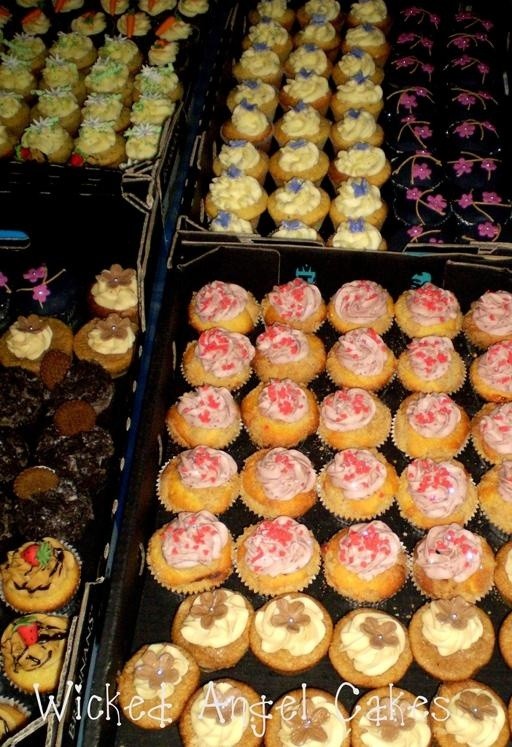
[0,0,212,172]
[204,1,511,251]
[0,259,143,747]
[115,279,511,746]
